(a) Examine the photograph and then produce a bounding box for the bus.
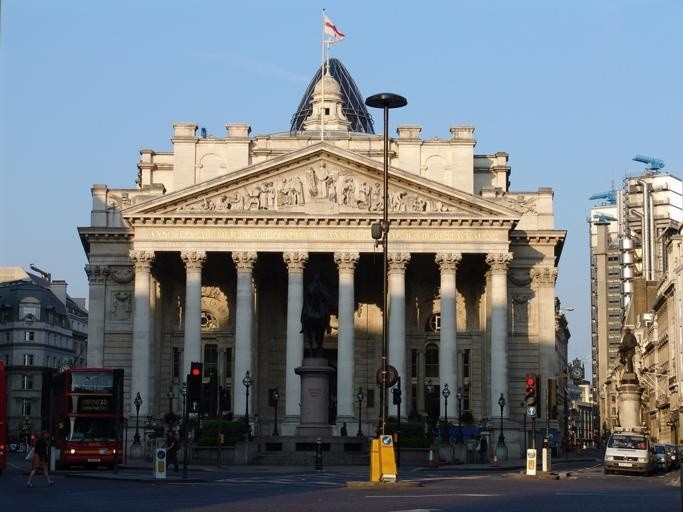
[0,360,8,471]
[62,368,124,469]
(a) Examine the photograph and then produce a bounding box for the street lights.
[456,387,464,445]
[243,370,251,430]
[426,378,434,468]
[133,392,141,446]
[441,384,452,442]
[273,390,280,437]
[167,387,173,415]
[357,387,364,438]
[180,383,189,472]
[497,394,506,447]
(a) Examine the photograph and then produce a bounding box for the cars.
[652,443,683,470]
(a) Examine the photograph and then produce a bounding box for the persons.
[480,436,487,464]
[162,431,179,472]
[560,436,569,457]
[179,157,458,214]
[26,430,57,487]
[341,422,347,436]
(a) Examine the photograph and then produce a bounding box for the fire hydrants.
[315,437,323,469]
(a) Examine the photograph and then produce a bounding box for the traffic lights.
[392,389,401,405]
[524,374,541,418]
[189,361,203,391]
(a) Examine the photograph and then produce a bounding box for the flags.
[324,15,345,41]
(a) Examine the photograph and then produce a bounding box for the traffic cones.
[428,444,435,466]
[490,452,501,467]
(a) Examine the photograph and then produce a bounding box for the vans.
[604,432,654,474]
